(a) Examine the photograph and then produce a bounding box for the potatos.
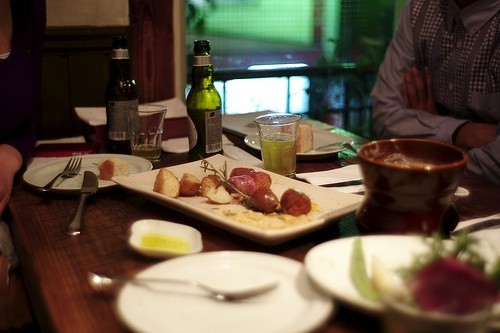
[154,168,233,204]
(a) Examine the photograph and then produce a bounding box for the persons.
[368,0,500,185]
[0,0,48,333]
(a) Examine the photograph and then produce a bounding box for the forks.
[40,156,83,192]
[85,273,273,305]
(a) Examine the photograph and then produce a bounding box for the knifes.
[66,170,98,236]
[318,179,362,187]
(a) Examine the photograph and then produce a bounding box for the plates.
[128,219,204,259]
[22,154,153,191]
[117,250,334,333]
[244,127,352,161]
[303,235,500,326]
[110,153,367,247]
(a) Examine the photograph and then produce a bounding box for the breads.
[98,158,126,180]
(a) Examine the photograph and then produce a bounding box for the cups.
[253,112,301,179]
[126,103,168,169]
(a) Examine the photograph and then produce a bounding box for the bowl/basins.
[357,138,468,217]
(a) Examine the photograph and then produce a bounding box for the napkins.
[296,165,364,193]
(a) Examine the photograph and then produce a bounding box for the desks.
[8,134,499,333]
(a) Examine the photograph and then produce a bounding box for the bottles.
[185,39,224,163]
[105,34,139,155]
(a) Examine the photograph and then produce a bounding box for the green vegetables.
[350,233,500,310]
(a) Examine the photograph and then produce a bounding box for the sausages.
[225,167,311,215]
